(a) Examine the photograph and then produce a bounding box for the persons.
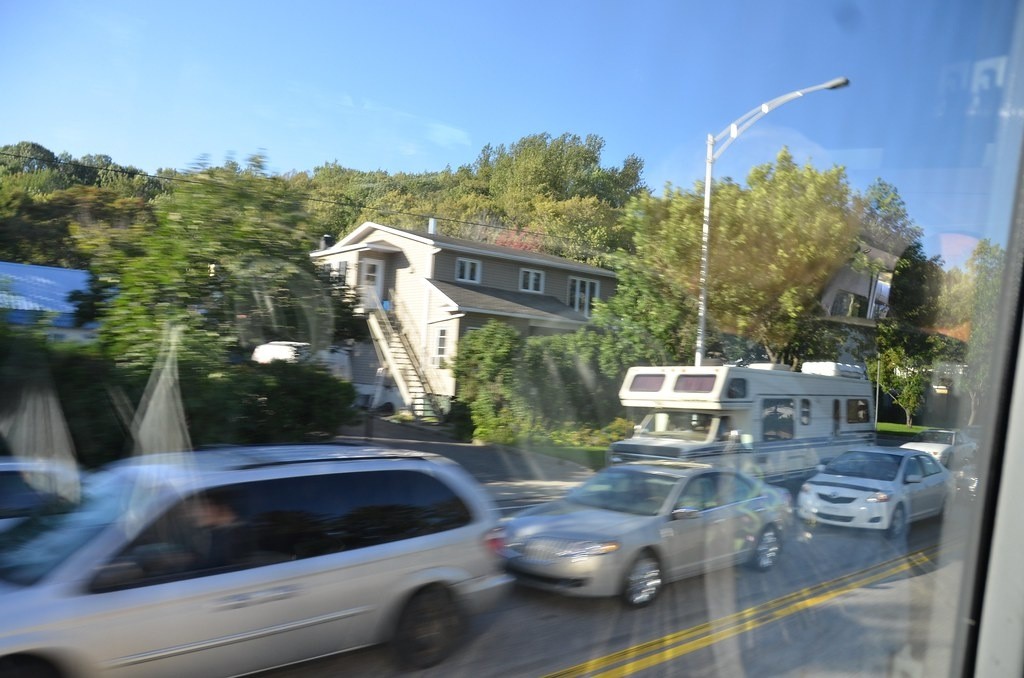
[969,66,1005,113]
[125,488,269,588]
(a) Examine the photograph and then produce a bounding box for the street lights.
[692,75,851,367]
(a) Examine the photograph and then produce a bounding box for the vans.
[0,443,518,678]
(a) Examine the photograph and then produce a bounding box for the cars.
[795,445,951,539]
[496,456,793,609]
[899,430,954,462]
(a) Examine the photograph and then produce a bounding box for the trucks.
[604,360,876,484]
